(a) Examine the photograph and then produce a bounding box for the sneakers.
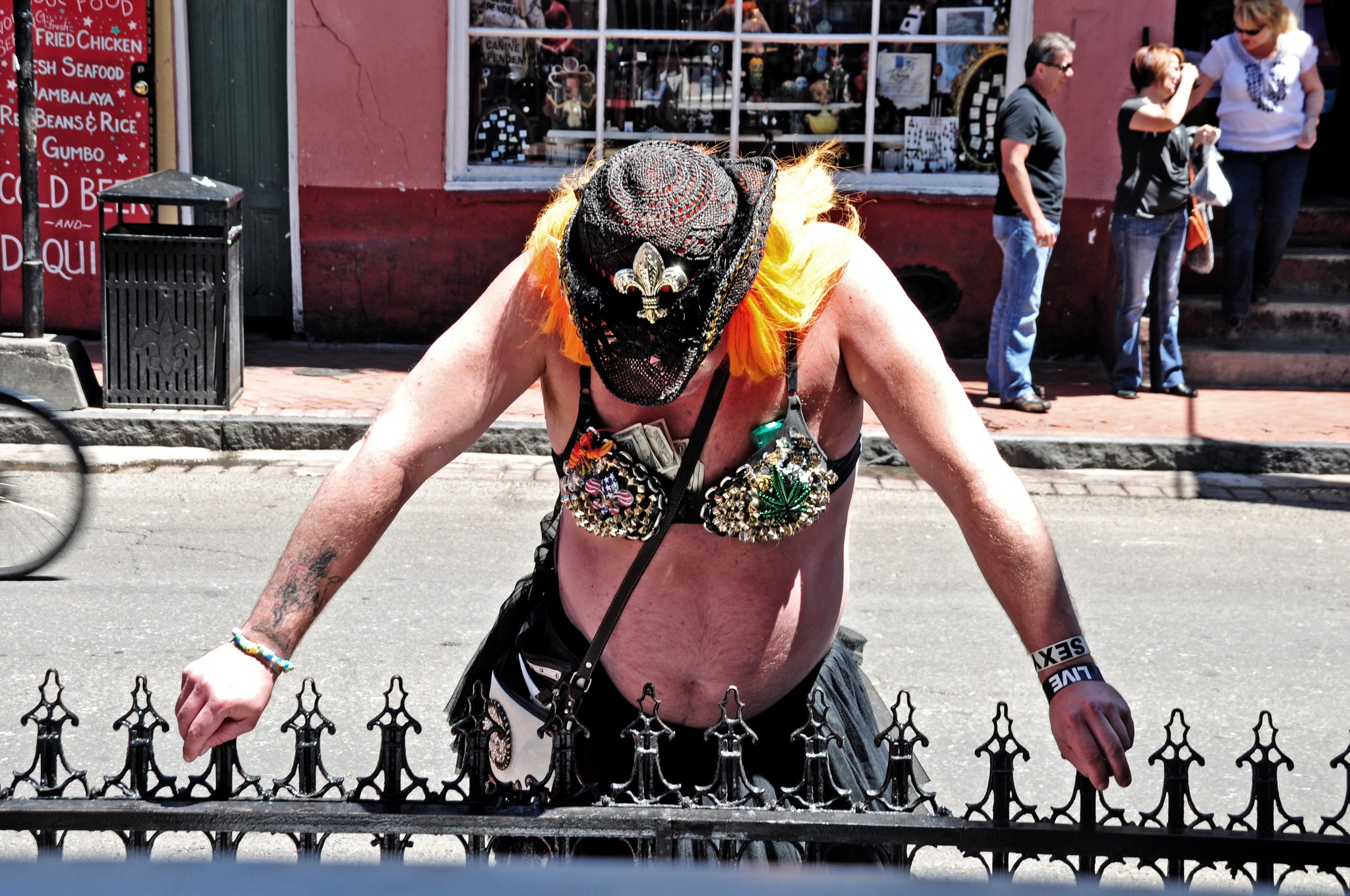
[1000,393,1051,411]
[987,386,1048,398]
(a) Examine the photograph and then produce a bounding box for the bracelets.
[1305,114,1320,126]
[231,629,295,676]
[1039,664,1103,703]
[1029,634,1090,675]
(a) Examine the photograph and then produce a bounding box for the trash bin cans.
[95,168,245,410]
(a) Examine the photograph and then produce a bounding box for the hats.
[559,140,778,407]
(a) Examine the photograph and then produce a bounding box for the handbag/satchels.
[1187,203,1215,275]
[1189,141,1233,207]
[1186,208,1208,250]
[457,628,590,807]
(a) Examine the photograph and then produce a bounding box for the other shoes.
[1224,316,1244,340]
[1251,288,1269,303]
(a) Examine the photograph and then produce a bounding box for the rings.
[1041,240,1046,245]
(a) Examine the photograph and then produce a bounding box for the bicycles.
[0,387,91,583]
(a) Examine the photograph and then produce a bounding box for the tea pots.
[806,106,839,134]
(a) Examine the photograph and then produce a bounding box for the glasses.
[1234,18,1267,35]
[1038,60,1073,72]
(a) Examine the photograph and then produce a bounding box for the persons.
[985,30,1076,412]
[1110,42,1223,399]
[174,141,1135,859]
[1185,0,1326,343]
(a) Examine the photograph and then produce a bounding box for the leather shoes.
[1151,383,1198,397]
[1117,387,1137,398]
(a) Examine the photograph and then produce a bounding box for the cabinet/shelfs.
[548,104,906,142]
